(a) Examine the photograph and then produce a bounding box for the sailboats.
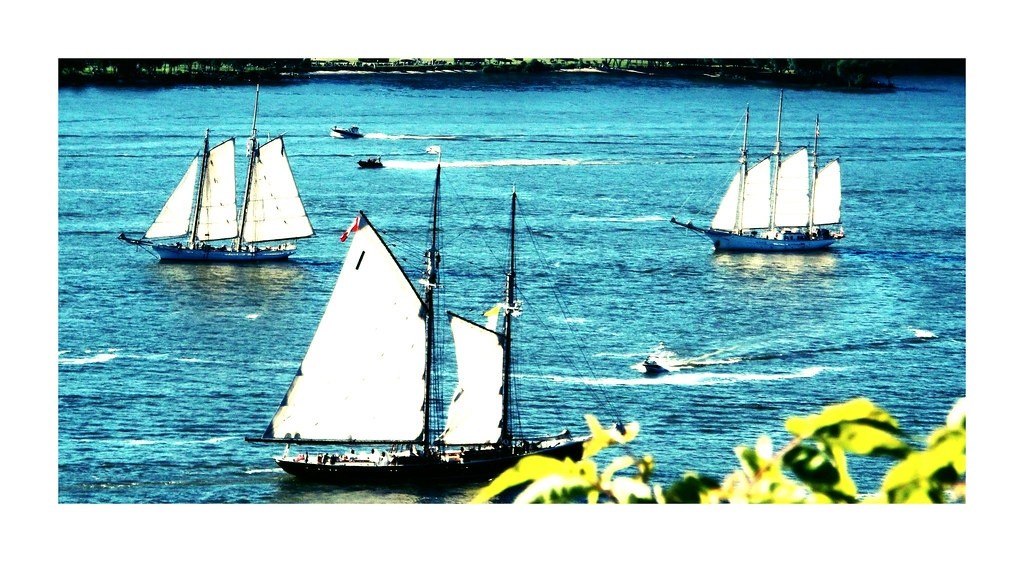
[243,145,628,493]
[669,88,846,252]
[116,83,315,264]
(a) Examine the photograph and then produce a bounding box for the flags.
[340,216,361,242]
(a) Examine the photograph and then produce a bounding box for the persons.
[153,240,293,254]
[740,227,842,240]
[294,445,465,464]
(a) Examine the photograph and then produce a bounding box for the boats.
[357,157,384,167]
[331,124,363,139]
[641,356,670,373]
